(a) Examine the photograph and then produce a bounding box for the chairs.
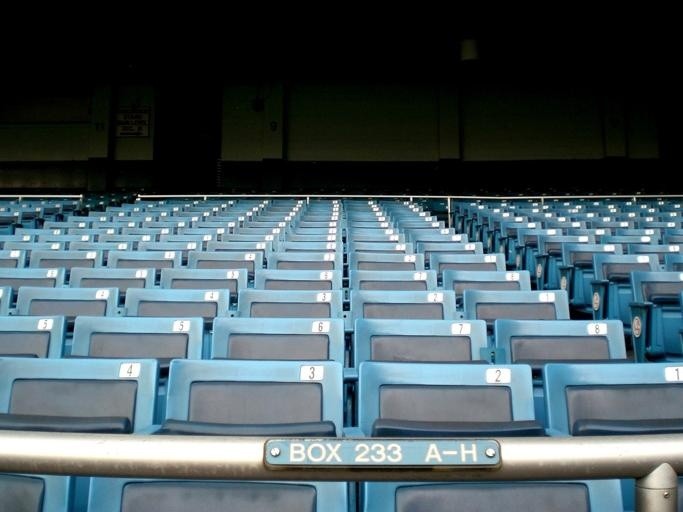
[0,194,682,512]
